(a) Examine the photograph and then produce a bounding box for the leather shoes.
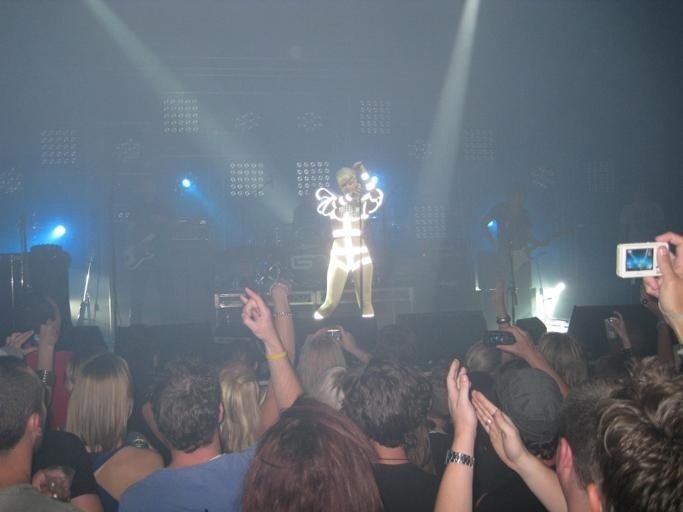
[312,311,324,320]
[360,313,377,319]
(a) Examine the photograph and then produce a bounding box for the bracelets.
[35,369,56,386]
[267,351,287,361]
[272,312,293,322]
[620,348,635,359]
[269,280,291,295]
[497,317,510,324]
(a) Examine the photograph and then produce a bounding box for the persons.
[312,366,350,411]
[12,293,76,431]
[597,232,683,512]
[538,331,590,387]
[218,278,296,455]
[61,350,164,511]
[607,310,636,362]
[464,338,516,378]
[487,326,571,512]
[119,288,304,512]
[0,367,85,512]
[370,323,423,366]
[639,287,674,372]
[243,397,383,512]
[435,357,624,512]
[314,161,384,319]
[0,356,103,512]
[65,341,110,394]
[480,185,545,322]
[0,317,59,407]
[339,352,442,512]
[223,338,261,375]
[298,325,377,392]
[426,369,458,476]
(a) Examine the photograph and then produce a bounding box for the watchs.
[443,449,475,467]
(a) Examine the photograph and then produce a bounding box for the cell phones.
[484,331,514,346]
[618,242,669,278]
[326,330,342,341]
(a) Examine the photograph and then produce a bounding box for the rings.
[486,420,491,425]
[642,299,648,304]
